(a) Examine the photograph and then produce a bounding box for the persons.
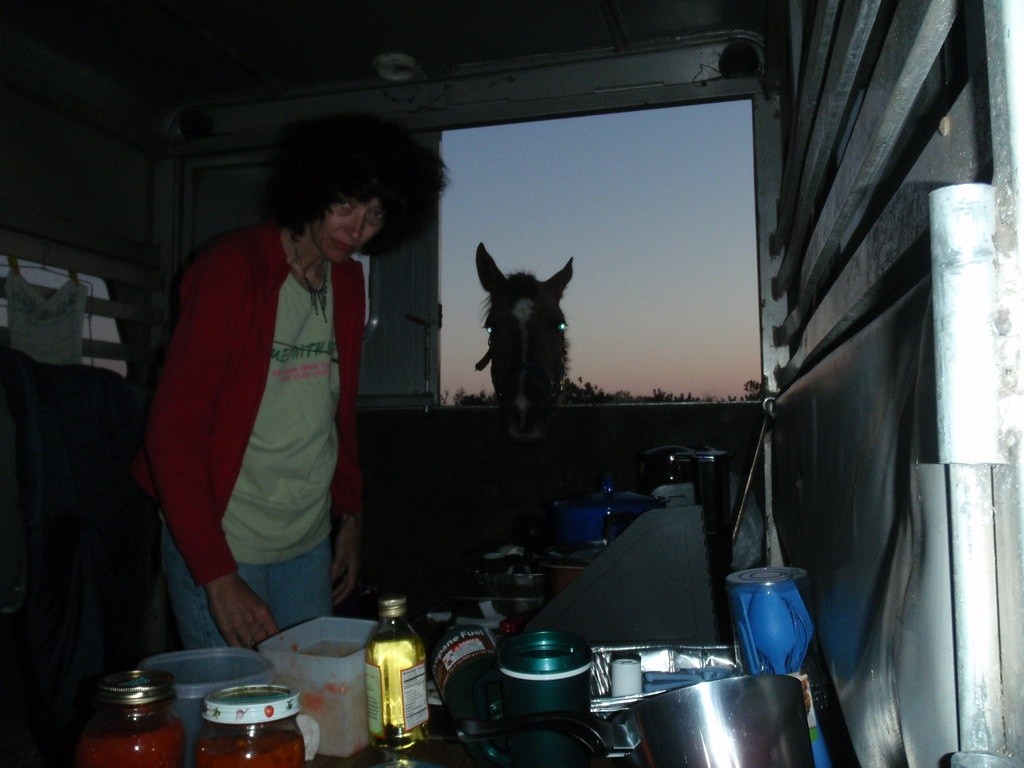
[131,111,446,653]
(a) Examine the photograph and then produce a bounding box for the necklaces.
[290,233,329,324]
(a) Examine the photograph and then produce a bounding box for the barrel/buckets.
[497,630,592,768]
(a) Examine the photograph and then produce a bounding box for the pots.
[453,673,816,768]
[552,473,672,544]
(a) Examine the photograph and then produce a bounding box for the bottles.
[364,596,429,759]
[725,566,831,673]
[429,625,505,742]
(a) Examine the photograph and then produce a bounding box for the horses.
[474,243,573,445]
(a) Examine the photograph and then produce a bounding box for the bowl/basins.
[483,571,545,592]
